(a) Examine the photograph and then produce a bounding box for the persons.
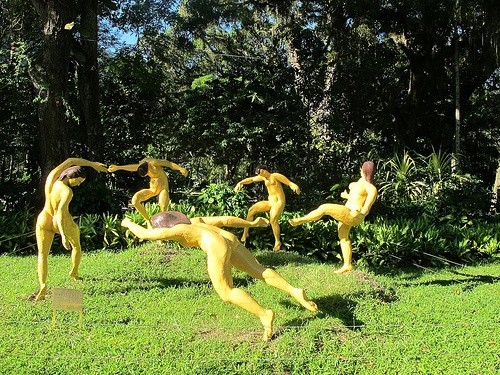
[233,164,301,252]
[108,156,189,229]
[287,161,377,275]
[120,210,318,343]
[34,157,108,301]
